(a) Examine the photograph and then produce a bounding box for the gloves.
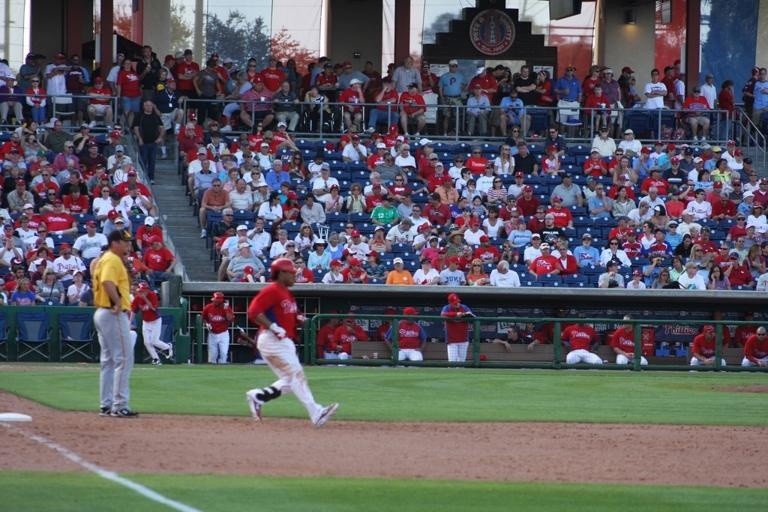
[295,312,304,323]
[267,322,286,341]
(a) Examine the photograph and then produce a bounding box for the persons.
[93,229,137,417]
[0,46,768,372]
[245,259,338,428]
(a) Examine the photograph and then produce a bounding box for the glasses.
[113,127,121,131]
[36,230,46,234]
[46,272,55,276]
[67,145,74,149]
[48,192,55,196]
[41,174,48,177]
[756,334,764,337]
[31,80,39,83]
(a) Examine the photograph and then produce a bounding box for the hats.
[52,198,62,203]
[106,228,135,243]
[402,306,419,317]
[0,117,165,279]
[0,48,767,99]
[209,290,225,303]
[701,323,716,335]
[756,326,766,335]
[621,314,633,322]
[447,292,459,306]
[178,118,767,280]
[385,308,396,317]
[325,309,355,320]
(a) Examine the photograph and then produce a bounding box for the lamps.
[626,9,636,24]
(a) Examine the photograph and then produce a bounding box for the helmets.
[269,257,297,276]
[135,281,150,293]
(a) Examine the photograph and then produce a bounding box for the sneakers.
[149,357,162,368]
[244,390,263,424]
[163,343,173,360]
[312,401,339,428]
[97,404,139,419]
